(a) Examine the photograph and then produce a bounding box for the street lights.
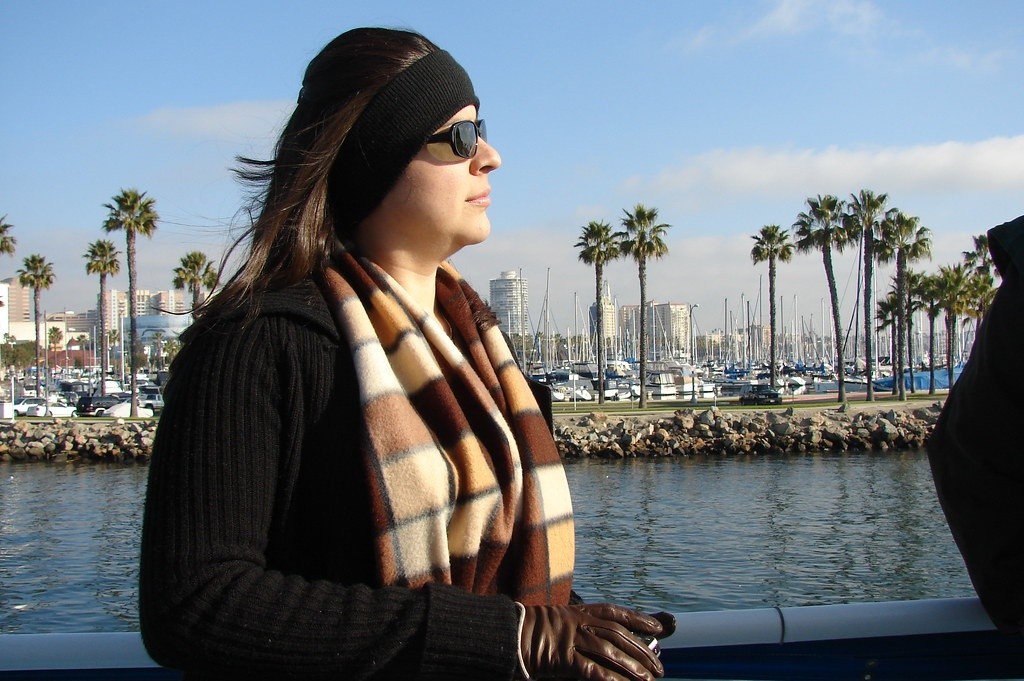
[690,304,699,402]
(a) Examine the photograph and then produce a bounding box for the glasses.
[427,119,488,159]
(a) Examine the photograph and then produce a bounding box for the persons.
[925,215,1024,652]
[138,27,677,681]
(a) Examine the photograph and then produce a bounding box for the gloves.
[515,602,677,681]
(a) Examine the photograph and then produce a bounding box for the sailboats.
[527,267,966,401]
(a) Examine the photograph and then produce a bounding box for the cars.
[76,396,123,417]
[137,394,164,410]
[26,402,80,418]
[738,384,782,406]
[42,373,165,405]
[23,384,37,397]
[14,397,46,416]
